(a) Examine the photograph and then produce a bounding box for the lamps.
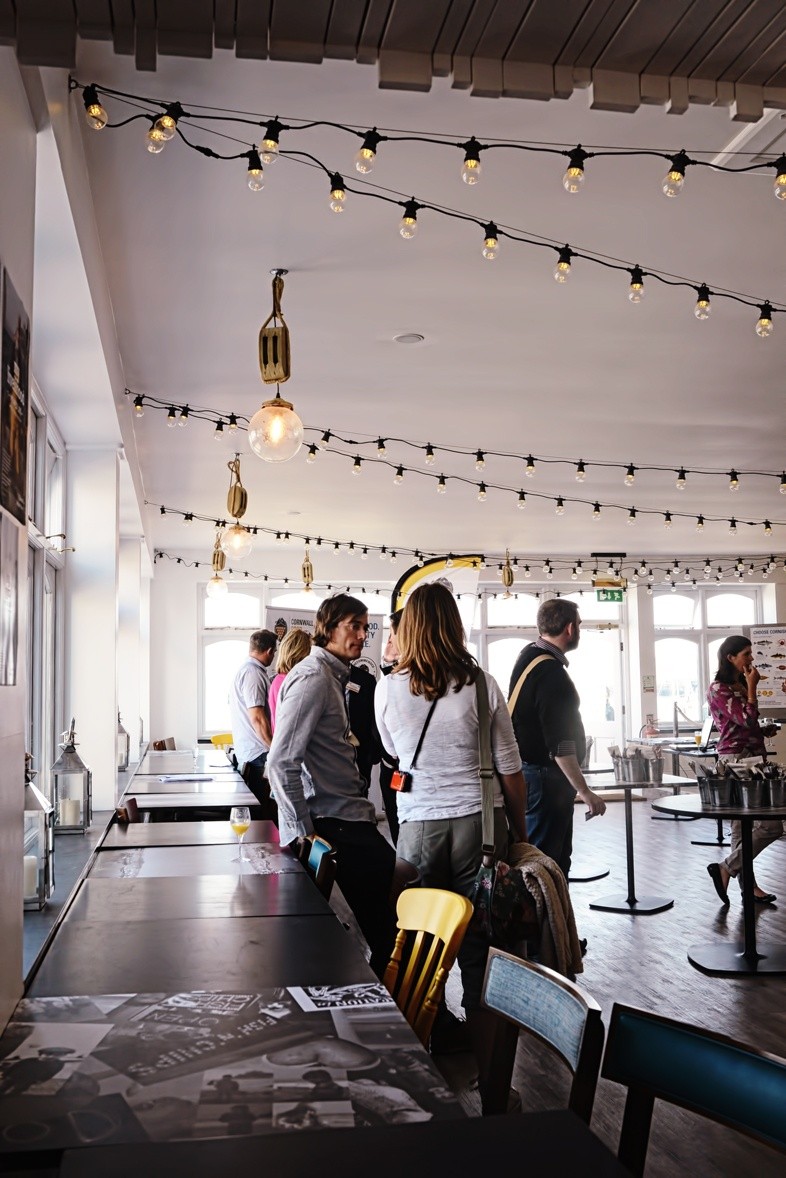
[248,268,305,463]
[296,548,316,607]
[222,453,253,561]
[207,533,229,598]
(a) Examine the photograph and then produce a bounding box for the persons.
[232,629,276,828]
[508,598,607,950]
[706,635,779,904]
[267,583,528,1118]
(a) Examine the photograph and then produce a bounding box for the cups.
[728,763,747,778]
[732,779,762,808]
[709,776,731,807]
[697,776,710,803]
[613,758,641,781]
[764,779,786,807]
[640,757,664,781]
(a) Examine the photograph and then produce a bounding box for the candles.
[24,856,37,896]
[60,798,80,825]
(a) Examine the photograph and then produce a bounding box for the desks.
[0,751,479,1178]
[569,737,786,978]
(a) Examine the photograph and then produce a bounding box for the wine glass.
[764,718,775,747]
[230,807,251,862]
[695,731,702,754]
[191,739,199,768]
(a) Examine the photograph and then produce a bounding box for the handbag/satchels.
[389,770,412,793]
[466,853,536,945]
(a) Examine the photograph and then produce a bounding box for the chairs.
[211,734,233,750]
[480,945,604,1128]
[300,835,338,902]
[602,1002,786,1178]
[383,887,475,1047]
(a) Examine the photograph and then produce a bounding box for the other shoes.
[430,1018,473,1056]
[482,1084,521,1120]
[706,863,731,906]
[741,890,776,904]
